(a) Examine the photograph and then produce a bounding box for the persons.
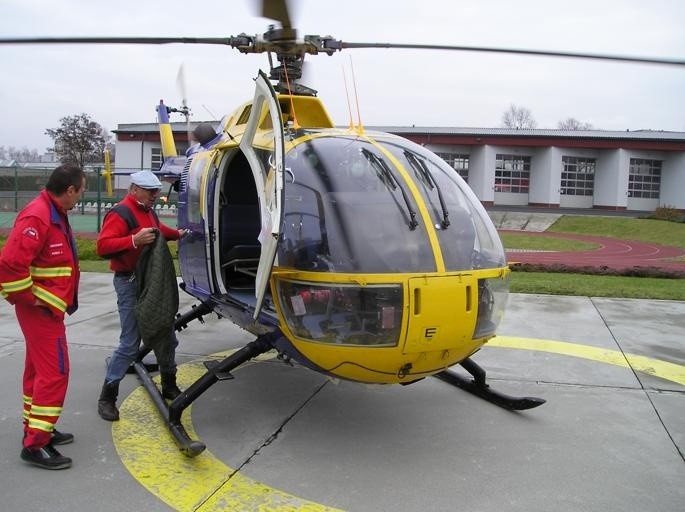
[0,165,85,470]
[96,170,188,421]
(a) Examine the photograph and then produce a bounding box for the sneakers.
[20,428,75,471]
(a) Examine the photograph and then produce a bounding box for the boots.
[159,367,184,401]
[97,378,120,422]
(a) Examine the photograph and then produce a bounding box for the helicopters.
[1,0,684,460]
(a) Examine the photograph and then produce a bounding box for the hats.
[129,169,164,190]
